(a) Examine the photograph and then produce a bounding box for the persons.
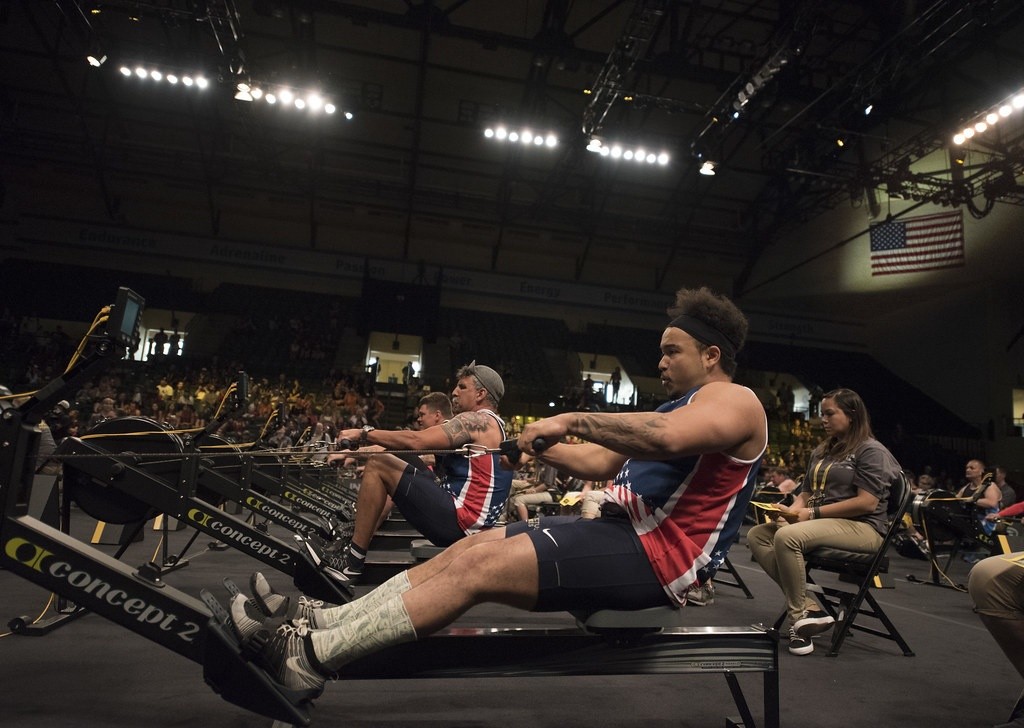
[967,550,1024,680]
[0,327,1024,607]
[228,288,768,709]
[746,388,902,657]
[304,358,514,588]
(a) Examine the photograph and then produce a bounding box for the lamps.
[89,3,104,17]
[690,140,720,179]
[712,39,806,130]
[117,57,215,91]
[235,73,340,115]
[127,8,144,24]
[865,104,884,126]
[835,135,847,148]
[86,35,113,69]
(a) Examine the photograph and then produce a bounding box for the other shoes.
[962,554,986,562]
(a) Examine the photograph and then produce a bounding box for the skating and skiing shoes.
[910,534,931,556]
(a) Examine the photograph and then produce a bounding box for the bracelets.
[813,506,819,518]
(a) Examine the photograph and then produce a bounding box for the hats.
[470,360,505,404]
[0,384,12,397]
[58,399,69,409]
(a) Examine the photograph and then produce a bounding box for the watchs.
[360,424,374,440]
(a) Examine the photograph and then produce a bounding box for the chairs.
[766,469,917,659]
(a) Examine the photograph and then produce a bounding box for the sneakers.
[892,539,906,554]
[687,584,716,606]
[304,532,367,588]
[342,500,357,521]
[252,572,330,636]
[228,593,340,700]
[795,608,835,636]
[897,533,911,547]
[328,517,372,546]
[788,624,814,655]
